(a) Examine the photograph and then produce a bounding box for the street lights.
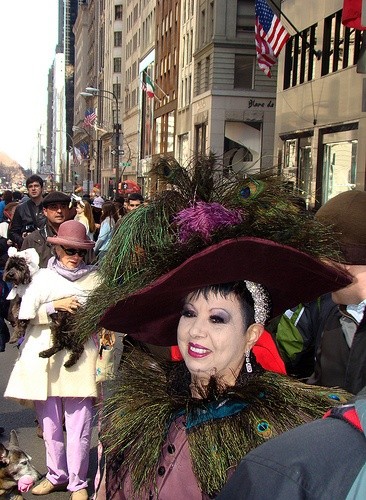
[72,125,96,183]
[79,87,124,202]
[46,145,63,192]
[55,129,78,195]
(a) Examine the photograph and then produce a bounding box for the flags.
[84,110,96,127]
[253,0,292,79]
[342,0,366,31]
[78,143,88,156]
[142,76,156,99]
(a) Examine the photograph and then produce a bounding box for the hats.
[112,196,125,204]
[91,196,105,209]
[313,190,366,265]
[47,220,95,249]
[100,236,354,347]
[43,191,72,204]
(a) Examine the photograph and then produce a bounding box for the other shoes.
[0,344,6,352]
[37,423,43,438]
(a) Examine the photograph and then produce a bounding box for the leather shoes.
[32,478,67,494]
[71,488,88,500]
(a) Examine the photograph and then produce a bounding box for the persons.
[0,176,148,500]
[94,189,366,500]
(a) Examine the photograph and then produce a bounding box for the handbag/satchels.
[95,329,116,381]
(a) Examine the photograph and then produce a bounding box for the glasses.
[60,245,87,257]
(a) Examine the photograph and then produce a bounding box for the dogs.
[1,257,32,347]
[0,427,44,500]
